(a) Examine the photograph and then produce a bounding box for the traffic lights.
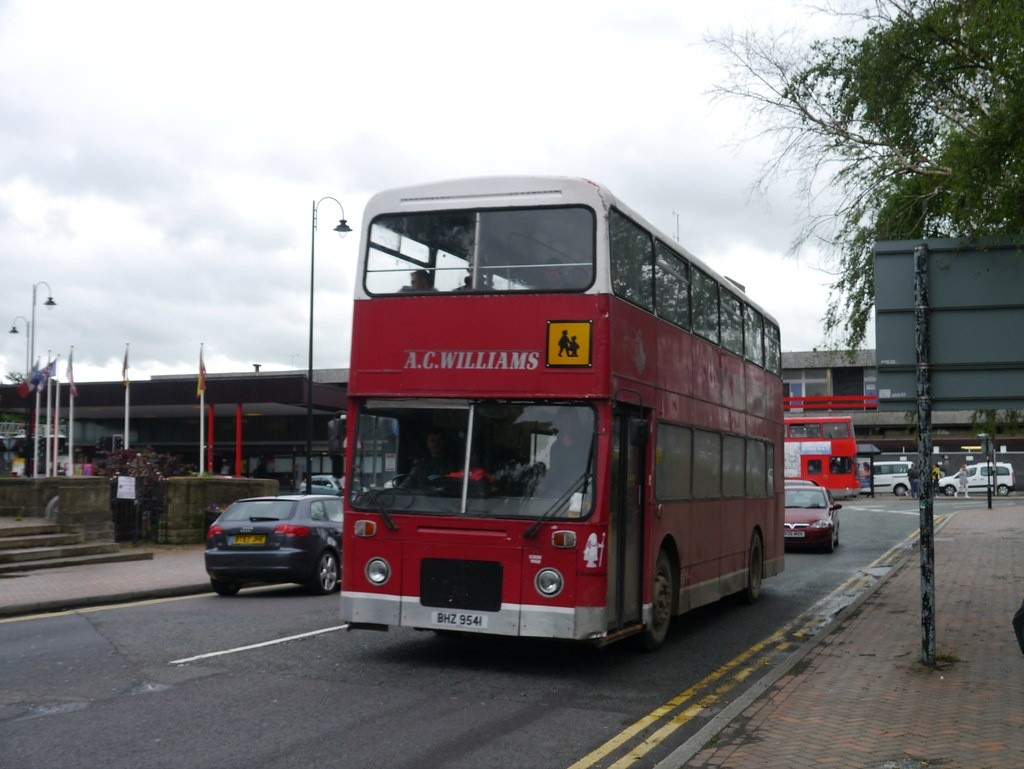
[112,435,123,453]
[94,435,103,456]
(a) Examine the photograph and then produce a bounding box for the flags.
[197,349,206,397]
[16,359,56,398]
[122,350,130,388]
[66,351,78,397]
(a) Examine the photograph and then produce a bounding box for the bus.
[783,416,862,496]
[327,176,788,644]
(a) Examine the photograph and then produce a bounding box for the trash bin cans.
[203,509,222,542]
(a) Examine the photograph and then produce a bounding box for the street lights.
[29,280,60,473]
[8,314,33,477]
[307,195,353,490]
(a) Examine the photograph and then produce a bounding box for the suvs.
[937,460,1016,498]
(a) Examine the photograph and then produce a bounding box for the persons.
[399,270,439,292]
[541,258,575,290]
[907,464,941,499]
[398,431,459,498]
[954,465,968,498]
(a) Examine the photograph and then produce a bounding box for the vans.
[859,461,916,497]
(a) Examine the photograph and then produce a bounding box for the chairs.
[785,495,796,505]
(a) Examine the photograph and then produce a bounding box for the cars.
[203,493,342,596]
[781,485,843,551]
[300,475,346,498]
